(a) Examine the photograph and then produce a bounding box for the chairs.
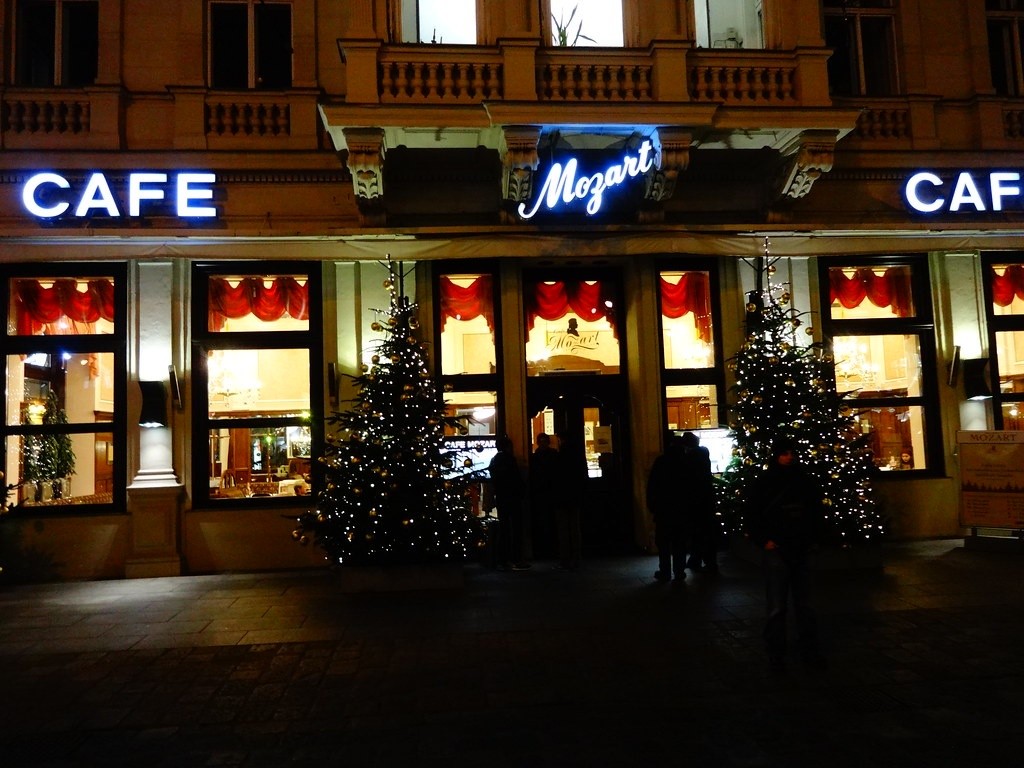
[219,468,237,498]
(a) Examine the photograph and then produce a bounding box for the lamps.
[962,357,994,402]
[137,381,168,428]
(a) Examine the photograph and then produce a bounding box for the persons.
[644,428,726,584]
[490,431,577,574]
[758,459,824,650]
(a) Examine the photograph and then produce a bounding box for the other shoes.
[675,572,686,580]
[799,648,828,670]
[654,571,671,581]
[497,563,505,572]
[512,561,535,570]
[697,564,718,573]
